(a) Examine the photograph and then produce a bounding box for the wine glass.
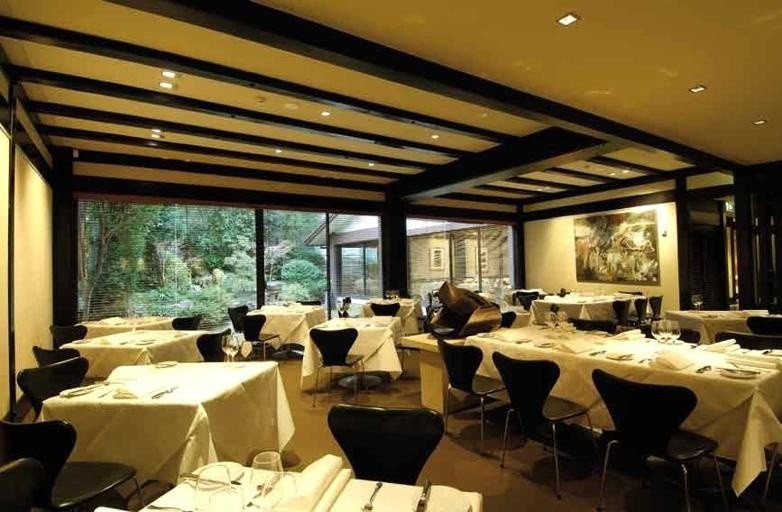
[650,319,681,344]
[544,311,569,333]
[337,303,353,319]
[385,290,400,300]
[221,335,240,368]
[194,465,234,512]
[691,293,704,313]
[247,451,283,512]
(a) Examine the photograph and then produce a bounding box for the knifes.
[416,478,431,512]
[180,472,242,486]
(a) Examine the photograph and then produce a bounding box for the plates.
[60,388,91,396]
[720,372,756,379]
[535,343,556,347]
[606,353,632,361]
[188,461,243,489]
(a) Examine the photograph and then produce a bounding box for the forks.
[360,482,383,512]
